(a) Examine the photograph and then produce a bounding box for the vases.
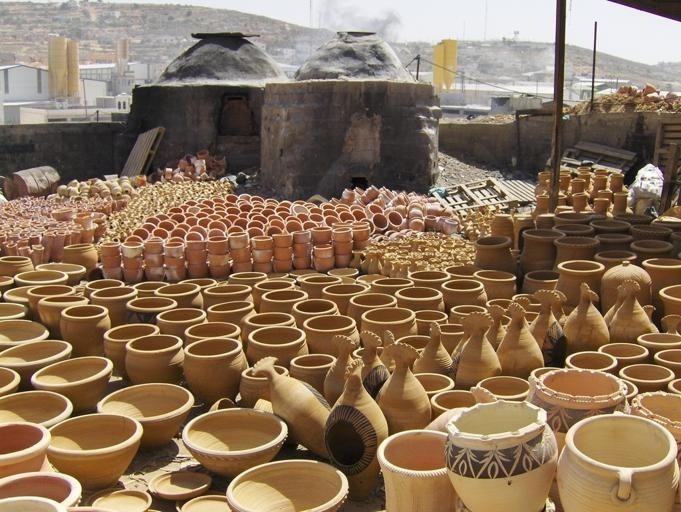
[446,399,558,512]
[615,82,681,108]
[560,409,679,511]
[0,436,350,510]
[1,150,680,435]
[322,361,387,502]
[376,427,455,512]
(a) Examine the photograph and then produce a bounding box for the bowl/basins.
[0,150,681,512]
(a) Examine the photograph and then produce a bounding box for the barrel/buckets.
[2,166,60,201]
